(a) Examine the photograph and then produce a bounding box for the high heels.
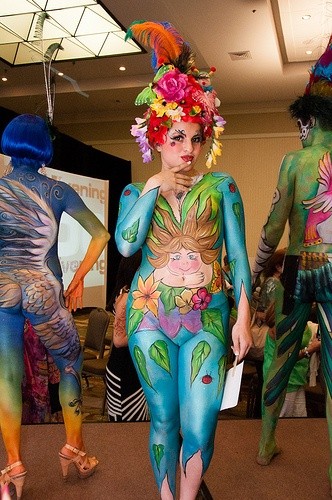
[58,442,98,480]
[0,461,29,500]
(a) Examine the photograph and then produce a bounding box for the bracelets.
[301,350,305,357]
[303,349,307,357]
[305,347,310,358]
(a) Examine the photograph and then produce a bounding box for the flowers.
[130,70,227,168]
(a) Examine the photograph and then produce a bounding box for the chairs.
[82,308,113,415]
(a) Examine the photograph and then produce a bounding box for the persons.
[0,113,111,500]
[18,248,325,421]
[251,93,332,483]
[114,100,252,500]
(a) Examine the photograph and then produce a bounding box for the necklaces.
[173,172,199,200]
